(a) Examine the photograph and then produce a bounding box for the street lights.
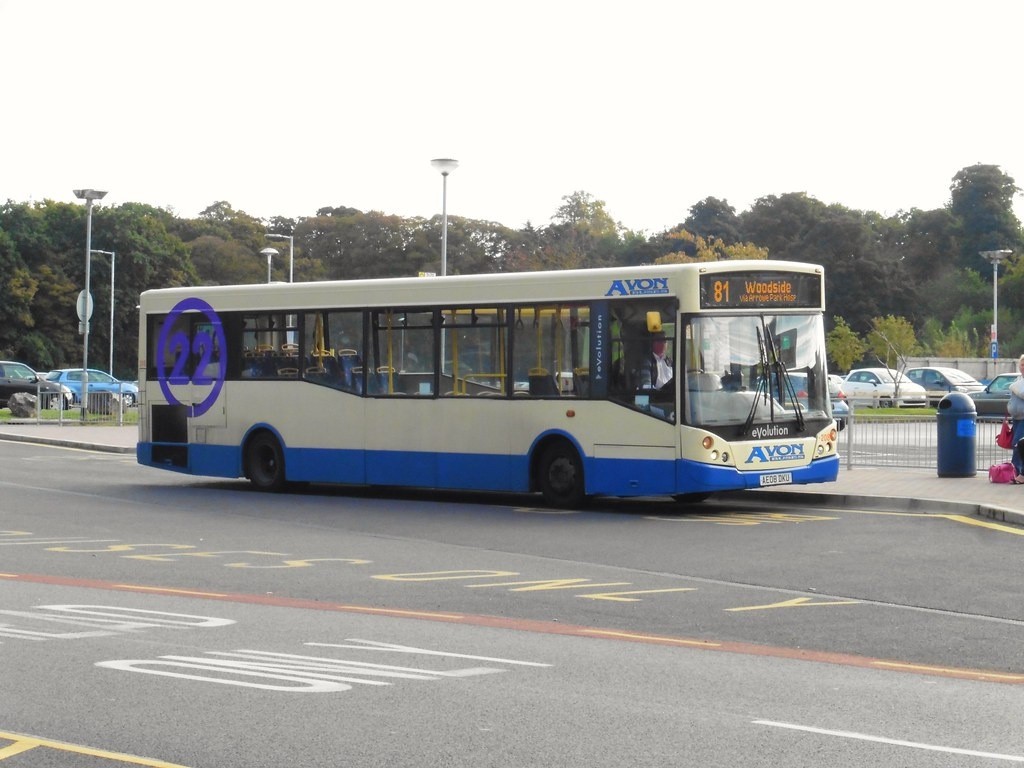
[978,249,1014,389]
[84,247,115,376]
[429,157,462,375]
[71,189,109,427]
[398,318,406,372]
[263,233,294,327]
[259,247,279,348]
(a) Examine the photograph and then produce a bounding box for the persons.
[649,331,673,388]
[1007,354,1024,482]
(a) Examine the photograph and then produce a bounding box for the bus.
[135,259,840,513]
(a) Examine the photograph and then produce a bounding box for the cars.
[840,367,926,409]
[904,365,988,407]
[754,373,850,432]
[45,367,139,407]
[968,372,1022,421]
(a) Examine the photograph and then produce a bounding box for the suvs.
[0,360,73,410]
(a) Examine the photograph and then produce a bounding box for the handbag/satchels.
[989,461,1018,483]
[994,409,1015,449]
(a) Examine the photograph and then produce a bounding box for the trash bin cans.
[936,394,977,477]
